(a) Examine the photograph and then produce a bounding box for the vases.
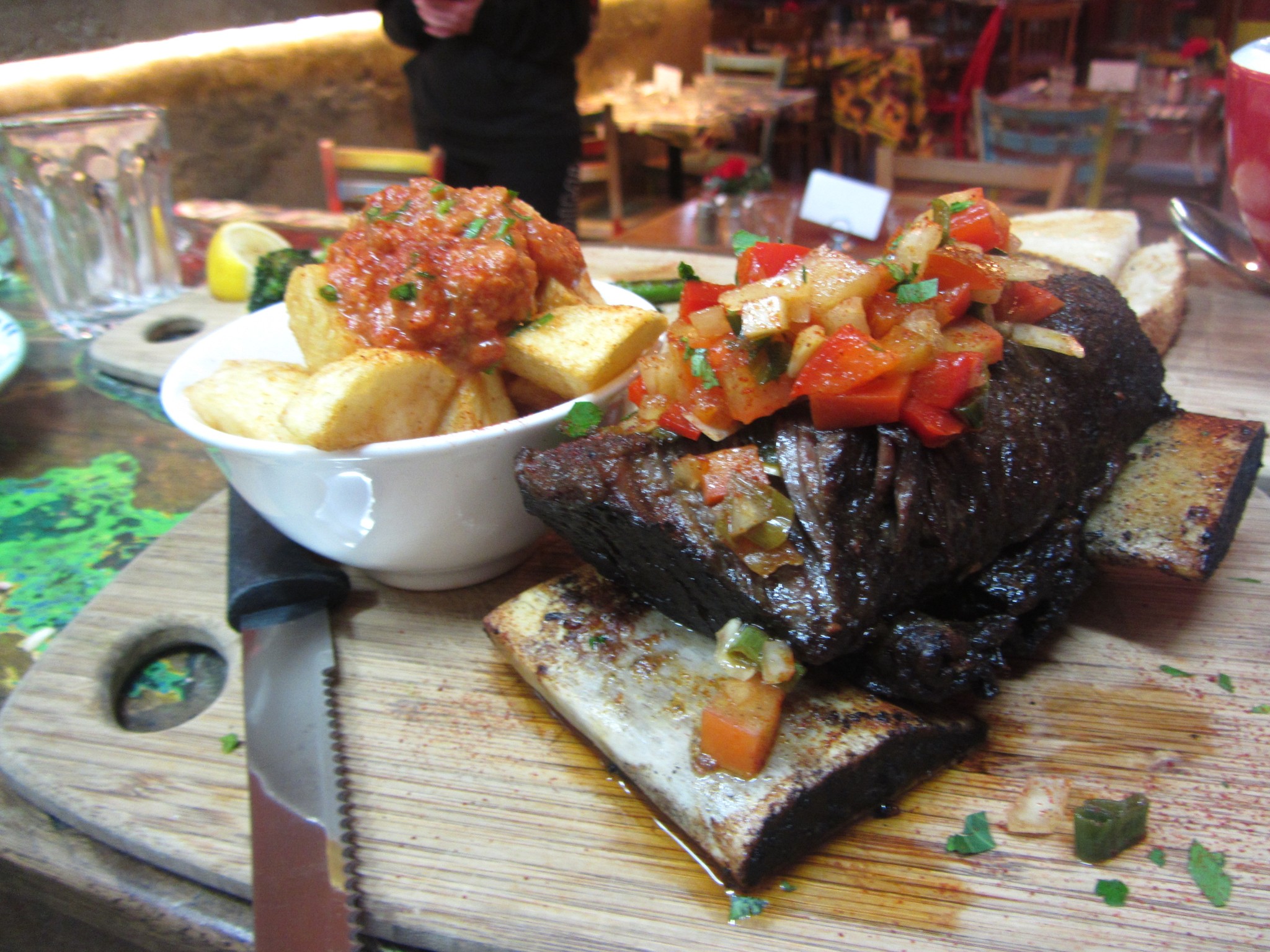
[714,189,755,255]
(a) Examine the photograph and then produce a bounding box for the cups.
[1050,65,1075,105]
[0,104,181,340]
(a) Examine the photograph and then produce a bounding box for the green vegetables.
[311,175,972,429]
[715,627,1234,917]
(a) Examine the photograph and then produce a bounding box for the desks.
[611,182,931,264]
[578,71,816,205]
[999,87,1220,185]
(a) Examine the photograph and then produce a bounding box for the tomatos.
[700,670,781,779]
[621,181,1060,440]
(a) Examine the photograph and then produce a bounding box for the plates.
[0,308,29,388]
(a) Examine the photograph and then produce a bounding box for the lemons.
[206,220,291,302]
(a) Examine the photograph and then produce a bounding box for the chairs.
[877,145,1070,219]
[317,137,445,212]
[702,46,787,159]
[973,89,1119,209]
[580,103,623,218]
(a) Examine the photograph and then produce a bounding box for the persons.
[376,1,600,246]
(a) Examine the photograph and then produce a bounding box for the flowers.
[703,149,778,201]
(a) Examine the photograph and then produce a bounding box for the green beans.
[609,277,704,302]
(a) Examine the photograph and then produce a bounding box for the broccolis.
[247,248,321,310]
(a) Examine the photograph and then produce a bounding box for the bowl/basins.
[159,281,666,592]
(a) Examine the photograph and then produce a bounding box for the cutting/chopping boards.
[0,288,1270,952]
[89,244,737,389]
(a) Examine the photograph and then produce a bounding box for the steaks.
[521,251,1267,721]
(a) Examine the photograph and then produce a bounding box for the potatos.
[193,264,667,453]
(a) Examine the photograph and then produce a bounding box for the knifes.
[226,486,363,952]
